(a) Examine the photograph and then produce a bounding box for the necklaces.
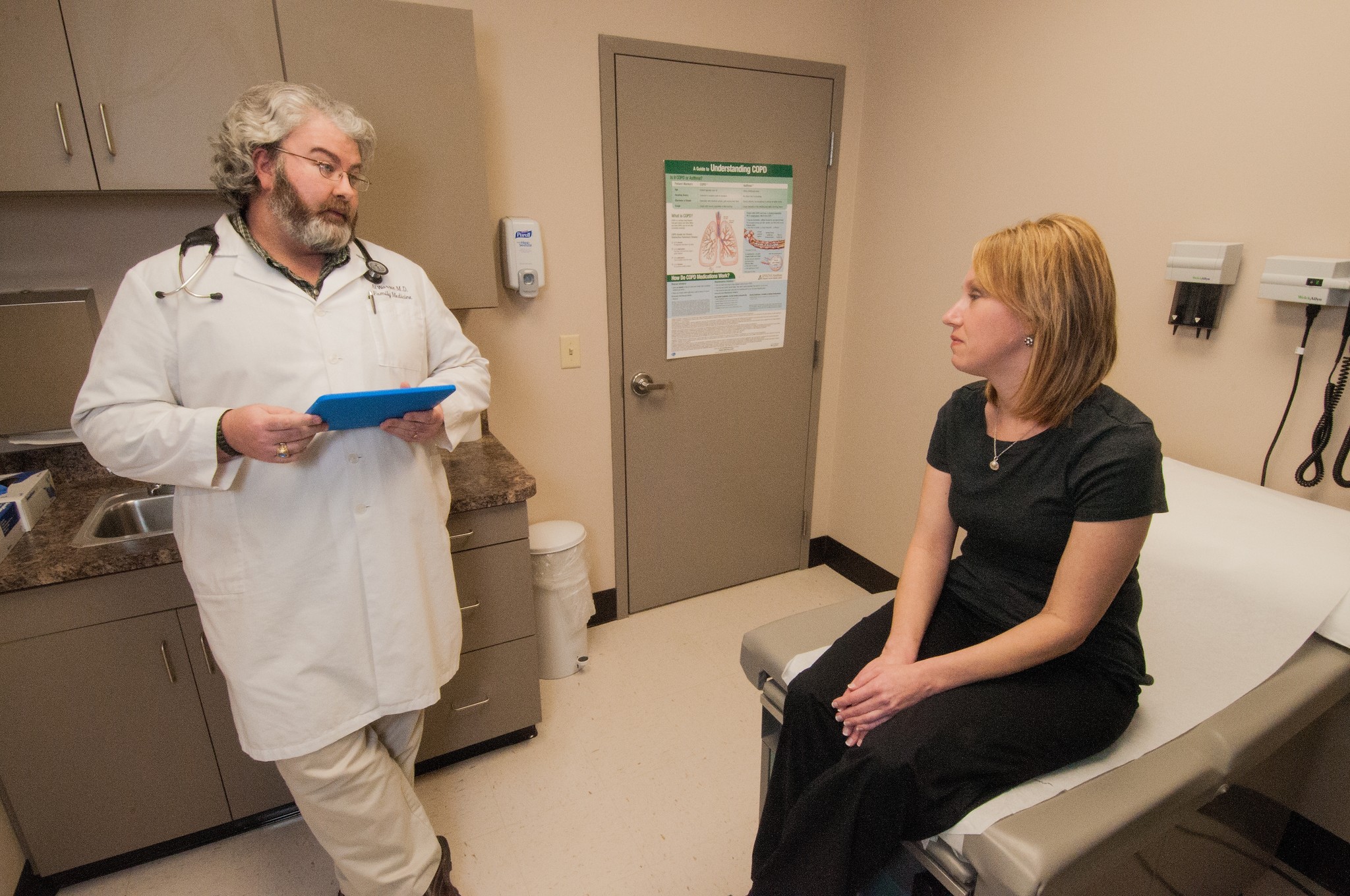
[991,405,1043,471]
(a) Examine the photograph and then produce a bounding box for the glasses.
[269,145,371,194]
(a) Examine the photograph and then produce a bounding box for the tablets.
[305,385,457,430]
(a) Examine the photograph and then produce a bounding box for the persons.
[732,213,1167,896]
[70,81,494,896]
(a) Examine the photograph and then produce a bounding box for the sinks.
[67,484,177,550]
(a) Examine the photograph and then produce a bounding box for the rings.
[412,432,419,439]
[276,443,290,458]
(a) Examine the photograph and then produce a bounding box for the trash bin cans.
[529,520,595,679]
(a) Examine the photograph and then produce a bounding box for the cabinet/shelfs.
[1,0,503,312]
[0,498,541,882]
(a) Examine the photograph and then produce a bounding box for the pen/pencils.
[368,291,376,314]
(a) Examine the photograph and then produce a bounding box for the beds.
[735,455,1350,896]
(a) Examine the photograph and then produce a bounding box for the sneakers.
[423,834,460,896]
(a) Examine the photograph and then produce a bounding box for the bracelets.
[216,409,244,456]
